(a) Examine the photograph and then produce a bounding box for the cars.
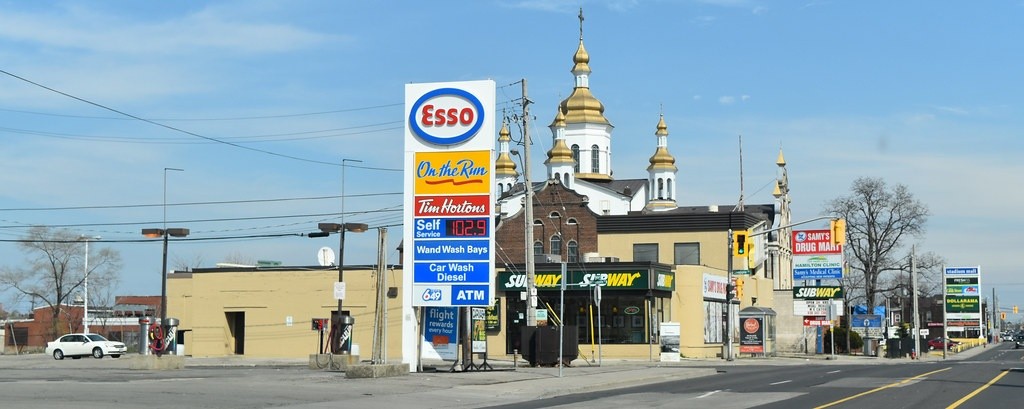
[1002,328,1024,348]
[45,332,128,360]
[928,335,958,350]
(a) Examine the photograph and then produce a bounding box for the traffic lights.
[1001,312,1005,319]
[726,284,736,303]
[735,231,749,256]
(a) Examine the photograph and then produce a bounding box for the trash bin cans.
[886,338,928,358]
[863,338,876,356]
[520,325,578,367]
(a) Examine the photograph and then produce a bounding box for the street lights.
[79,234,103,333]
[341,157,365,224]
[140,228,190,348]
[316,222,369,354]
[162,167,186,227]
